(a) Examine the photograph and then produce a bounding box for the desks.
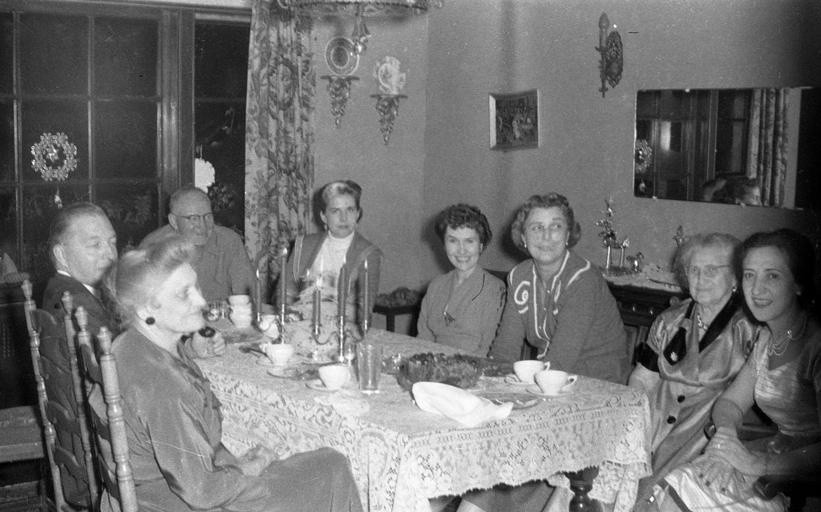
[607,261,688,328]
[374,303,421,338]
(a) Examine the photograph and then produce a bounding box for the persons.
[272,182,381,328]
[42,202,118,506]
[713,176,762,206]
[416,203,507,357]
[651,229,820,489]
[628,232,755,512]
[135,186,259,308]
[87,244,364,511]
[429,194,630,512]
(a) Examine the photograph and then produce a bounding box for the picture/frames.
[488,89,540,151]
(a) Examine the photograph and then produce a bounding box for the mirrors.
[633,87,802,210]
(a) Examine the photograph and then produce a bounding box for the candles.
[281,257,286,305]
[312,291,322,324]
[338,267,348,318]
[254,279,262,313]
[363,271,368,321]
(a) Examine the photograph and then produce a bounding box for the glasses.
[173,212,214,224]
[683,264,730,278]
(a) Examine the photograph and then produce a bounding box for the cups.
[318,365,351,387]
[356,341,382,391]
[265,343,294,365]
[535,370,578,394]
[228,295,249,306]
[512,360,550,381]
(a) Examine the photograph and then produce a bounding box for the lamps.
[273,0,446,54]
[635,140,654,193]
[595,12,623,98]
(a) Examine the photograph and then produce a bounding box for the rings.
[717,443,720,447]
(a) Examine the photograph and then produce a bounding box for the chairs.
[22,278,99,512]
[74,306,139,512]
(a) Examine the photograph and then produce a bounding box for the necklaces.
[767,313,808,356]
[695,311,708,332]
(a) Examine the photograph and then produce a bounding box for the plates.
[266,366,292,378]
[255,357,270,367]
[505,374,534,386]
[306,378,340,392]
[525,386,574,397]
